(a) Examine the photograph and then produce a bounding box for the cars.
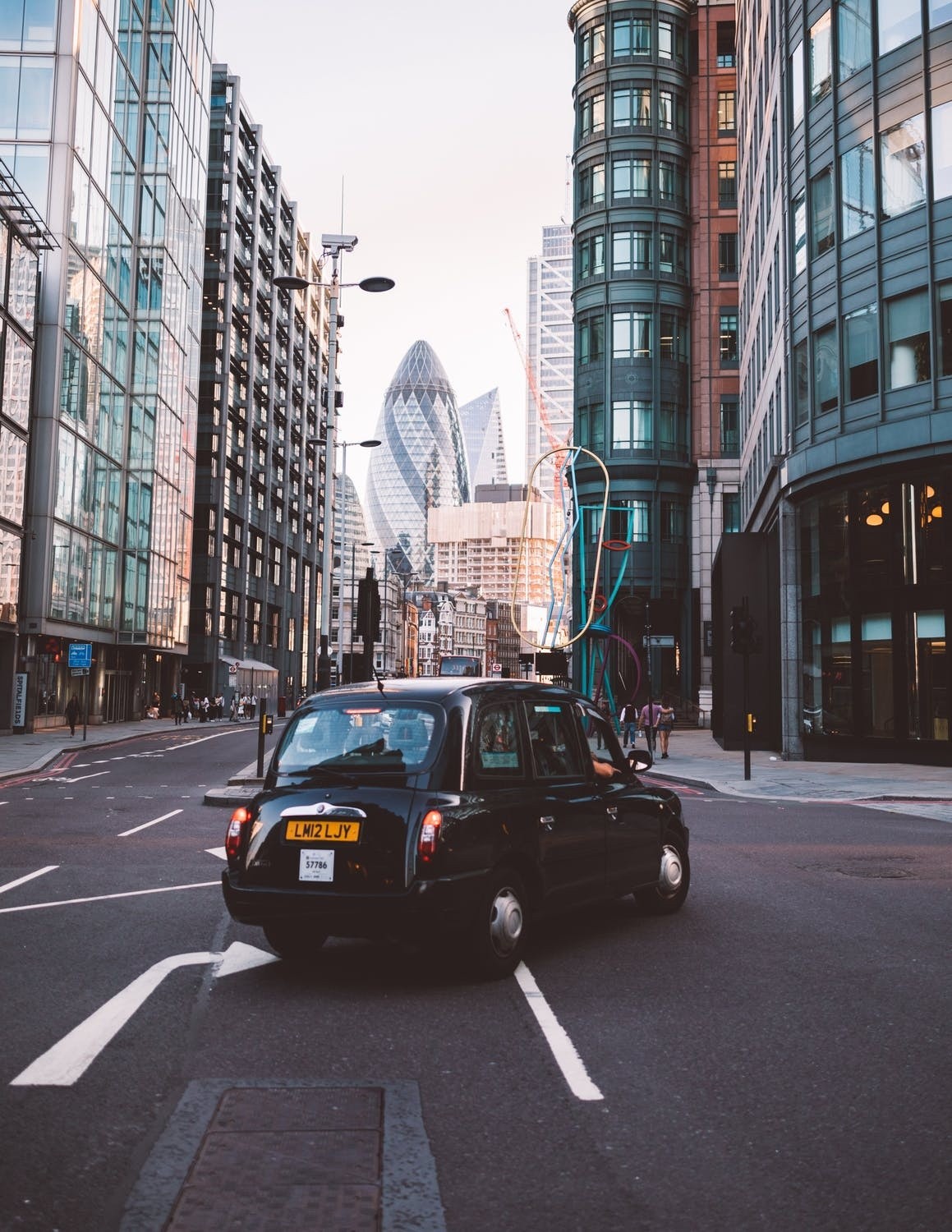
[219,674,694,984]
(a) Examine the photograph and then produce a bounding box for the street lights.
[334,538,448,688]
[271,273,396,699]
[306,436,382,684]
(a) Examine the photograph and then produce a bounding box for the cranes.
[503,308,573,512]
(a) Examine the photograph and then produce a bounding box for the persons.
[596,700,612,750]
[229,692,259,721]
[65,694,83,737]
[556,716,623,779]
[150,690,160,708]
[618,700,640,748]
[172,690,225,725]
[639,696,674,759]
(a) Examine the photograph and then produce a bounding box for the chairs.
[531,738,560,777]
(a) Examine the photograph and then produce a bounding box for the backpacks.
[661,712,672,725]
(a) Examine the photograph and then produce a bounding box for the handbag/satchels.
[188,712,192,720]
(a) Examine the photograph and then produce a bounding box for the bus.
[436,655,483,677]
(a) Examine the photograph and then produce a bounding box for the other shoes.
[661,752,669,759]
[631,744,635,749]
[623,744,627,748]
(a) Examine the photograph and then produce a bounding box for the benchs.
[296,718,427,765]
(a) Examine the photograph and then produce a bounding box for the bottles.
[638,729,642,738]
